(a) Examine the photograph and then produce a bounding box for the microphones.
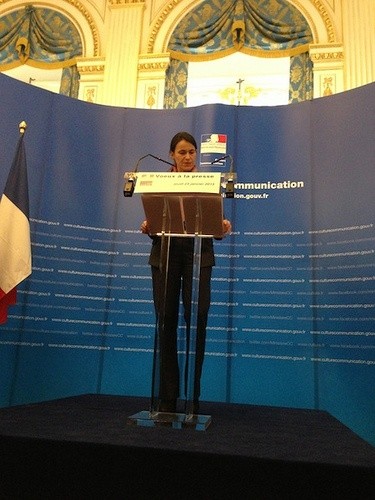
[135,154,176,172]
[211,155,233,172]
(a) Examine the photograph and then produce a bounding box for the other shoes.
[160,400,178,412]
[184,401,201,415]
